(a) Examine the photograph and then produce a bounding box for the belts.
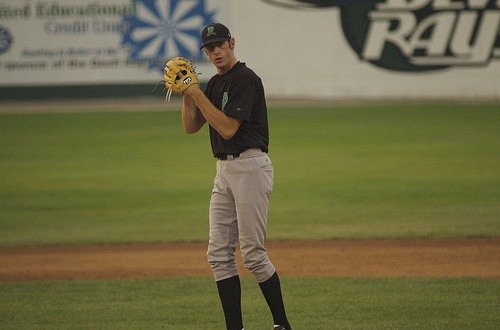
[218,154,239,160]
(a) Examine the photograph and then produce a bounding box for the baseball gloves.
[162,56,199,96]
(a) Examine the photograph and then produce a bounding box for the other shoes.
[273,324,286,330]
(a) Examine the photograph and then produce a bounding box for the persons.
[169,22,291,330]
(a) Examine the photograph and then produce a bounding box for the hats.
[199,23,231,50]
